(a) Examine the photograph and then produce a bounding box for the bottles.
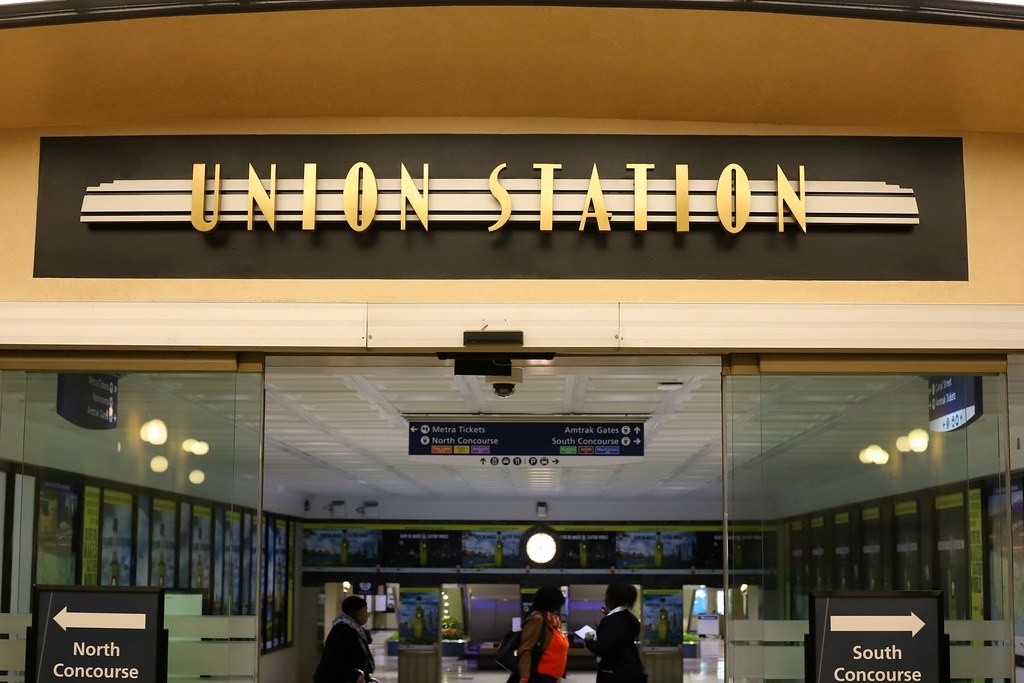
[654,532,663,569]
[414,596,425,641]
[796,535,958,619]
[658,599,668,642]
[111,518,282,613]
[579,534,588,567]
[494,531,504,569]
[339,530,350,566]
[420,532,428,566]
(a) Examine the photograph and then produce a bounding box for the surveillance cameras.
[485,366,524,398]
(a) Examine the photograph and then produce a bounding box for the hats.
[342,596,367,616]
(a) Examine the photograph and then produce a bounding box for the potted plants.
[385,616,472,657]
[681,632,701,658]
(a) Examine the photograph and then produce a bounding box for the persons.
[584,584,648,683]
[312,596,375,683]
[506,586,569,683]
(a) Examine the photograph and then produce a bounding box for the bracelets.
[520,677,528,680]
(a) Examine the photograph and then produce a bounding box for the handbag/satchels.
[495,611,546,676]
